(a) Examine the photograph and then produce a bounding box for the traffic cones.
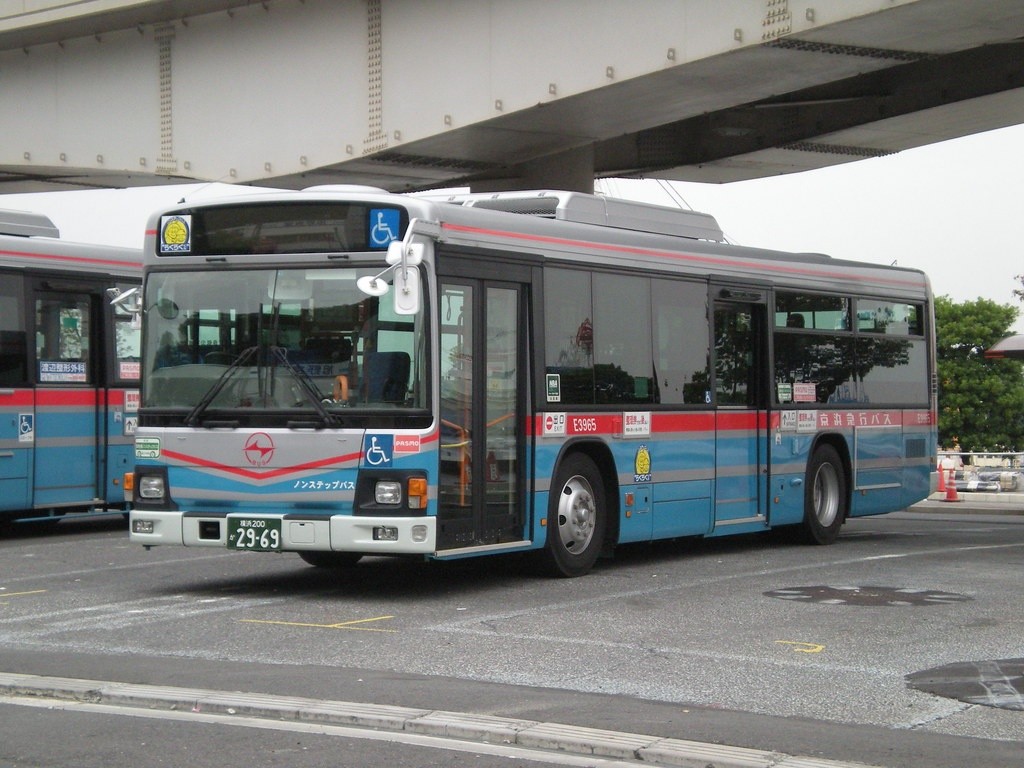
[937,460,945,496]
[939,467,961,502]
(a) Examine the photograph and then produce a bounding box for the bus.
[0,209,148,531]
[128,183,940,578]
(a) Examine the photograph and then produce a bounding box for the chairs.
[353,352,411,404]
[295,340,352,364]
[0,353,25,386]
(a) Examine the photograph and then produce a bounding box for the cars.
[937,450,965,480]
